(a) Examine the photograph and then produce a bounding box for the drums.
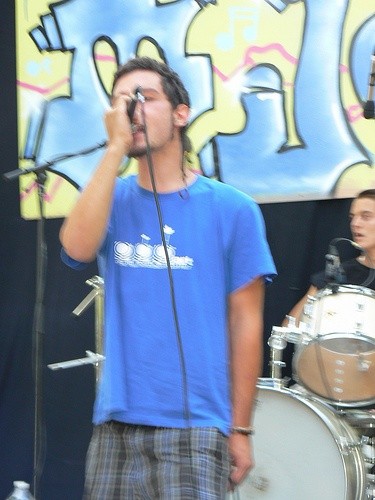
[291,283,375,407]
[225,379,366,499]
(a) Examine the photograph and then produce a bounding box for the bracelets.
[230,425,255,435]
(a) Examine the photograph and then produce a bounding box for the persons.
[58,56,278,500]
[281,189,375,328]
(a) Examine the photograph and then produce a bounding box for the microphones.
[326,245,341,293]
[128,88,139,123]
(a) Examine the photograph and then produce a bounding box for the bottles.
[7,479,38,500]
